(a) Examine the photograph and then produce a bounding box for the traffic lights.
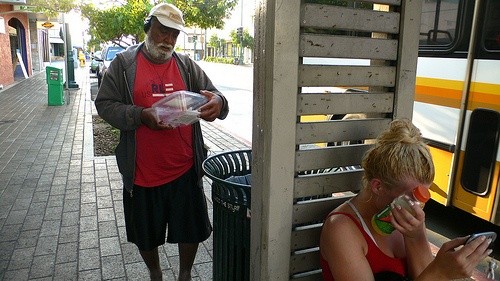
[193,36,196,43]
[237,28,239,37]
[240,27,243,36]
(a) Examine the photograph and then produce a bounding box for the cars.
[90,50,103,74]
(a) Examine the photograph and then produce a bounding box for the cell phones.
[464,232,497,247]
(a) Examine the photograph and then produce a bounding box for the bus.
[301,0,500,228]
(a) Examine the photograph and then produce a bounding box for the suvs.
[94,45,128,90]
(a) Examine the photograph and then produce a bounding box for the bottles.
[371,186,430,237]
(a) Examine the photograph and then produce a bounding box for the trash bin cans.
[202,149,251,281]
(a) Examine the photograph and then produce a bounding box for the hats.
[148,3,188,35]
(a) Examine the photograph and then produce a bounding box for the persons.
[94,3,229,281]
[320,117,493,281]
[85,50,90,62]
